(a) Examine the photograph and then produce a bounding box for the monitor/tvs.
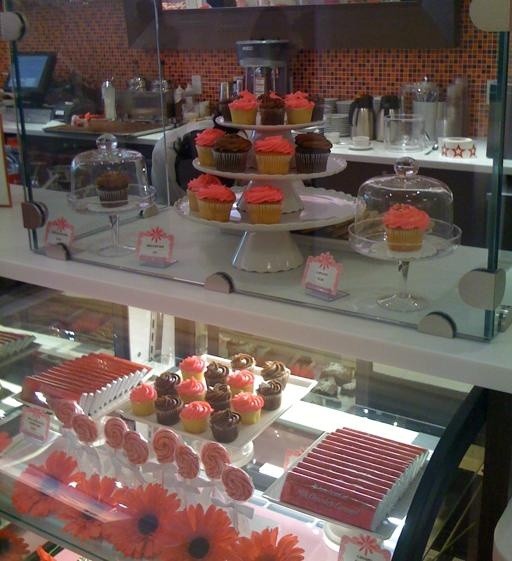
[4,51,58,108]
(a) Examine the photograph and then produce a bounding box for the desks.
[1,100,512,247]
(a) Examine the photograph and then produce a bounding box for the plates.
[175,186,363,233]
[349,145,373,152]
[192,158,347,184]
[318,95,387,139]
[214,115,325,136]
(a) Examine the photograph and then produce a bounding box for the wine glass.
[68,185,158,259]
[348,217,462,313]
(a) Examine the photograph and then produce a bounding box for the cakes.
[186,90,332,225]
[94,171,129,208]
[382,203,431,253]
[129,352,292,444]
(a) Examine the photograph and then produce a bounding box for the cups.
[219,80,243,105]
[325,132,340,145]
[353,136,370,148]
[384,78,465,160]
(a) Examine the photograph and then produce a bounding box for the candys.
[55,398,256,503]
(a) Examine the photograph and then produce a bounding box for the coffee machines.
[235,41,292,101]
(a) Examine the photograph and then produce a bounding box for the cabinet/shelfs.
[0,167,512,561]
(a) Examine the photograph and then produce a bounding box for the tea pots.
[375,95,403,143]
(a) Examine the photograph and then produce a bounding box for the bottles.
[102,81,117,122]
[126,77,168,92]
[175,83,208,125]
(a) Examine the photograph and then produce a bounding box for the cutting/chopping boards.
[45,122,174,137]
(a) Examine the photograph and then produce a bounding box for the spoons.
[425,143,437,157]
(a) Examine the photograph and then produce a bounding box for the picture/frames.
[121,0,463,57]
